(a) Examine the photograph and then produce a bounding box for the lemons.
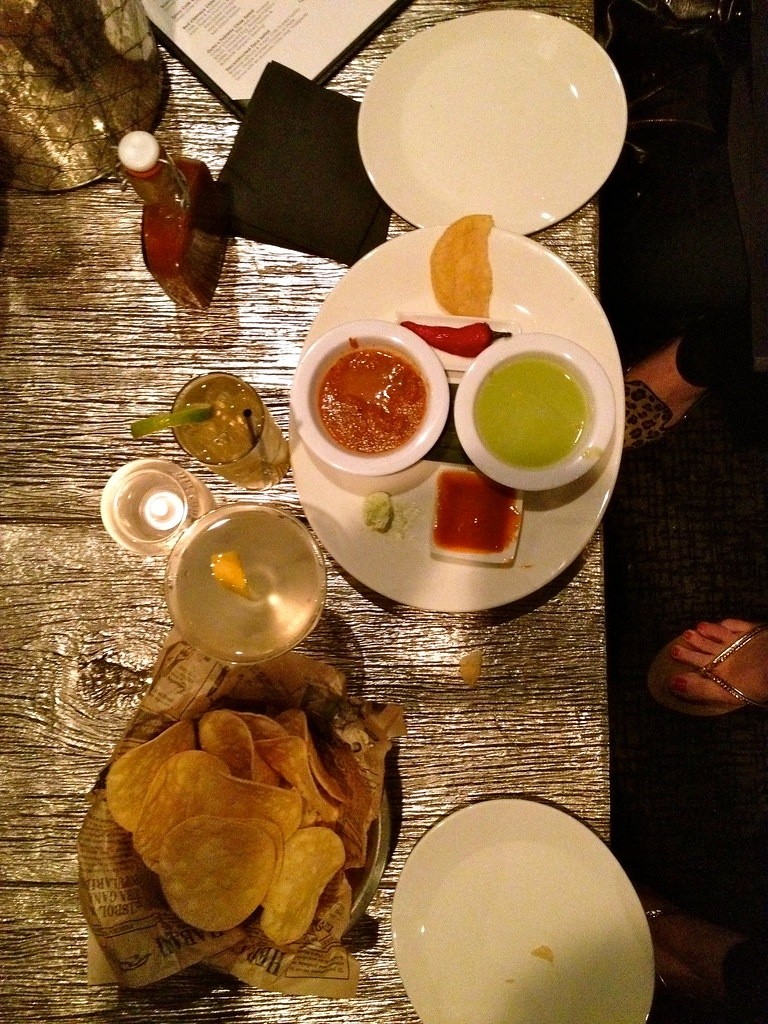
[130,403,212,439]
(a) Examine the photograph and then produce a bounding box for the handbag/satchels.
[594,0,749,197]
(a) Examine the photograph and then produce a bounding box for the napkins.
[197,58,392,268]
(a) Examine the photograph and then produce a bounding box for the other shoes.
[623,340,691,451]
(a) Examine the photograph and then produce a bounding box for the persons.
[624,303,767,449]
[637,619,768,1023]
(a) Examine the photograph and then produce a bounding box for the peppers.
[400,321,512,358]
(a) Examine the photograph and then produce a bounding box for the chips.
[105,708,351,946]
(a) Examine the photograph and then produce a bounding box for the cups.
[163,502,326,666]
[98,461,216,557]
[169,371,290,494]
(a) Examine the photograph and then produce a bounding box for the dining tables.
[1,0,608,1024]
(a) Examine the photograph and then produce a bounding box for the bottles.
[115,129,231,311]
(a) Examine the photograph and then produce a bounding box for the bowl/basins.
[288,321,451,479]
[453,333,617,490]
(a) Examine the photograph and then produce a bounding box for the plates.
[391,800,654,1024]
[289,226,626,614]
[357,10,629,234]
[427,462,524,567]
[391,312,522,384]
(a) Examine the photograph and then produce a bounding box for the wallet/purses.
[194,60,384,268]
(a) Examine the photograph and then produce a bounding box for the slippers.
[648,620,768,713]
